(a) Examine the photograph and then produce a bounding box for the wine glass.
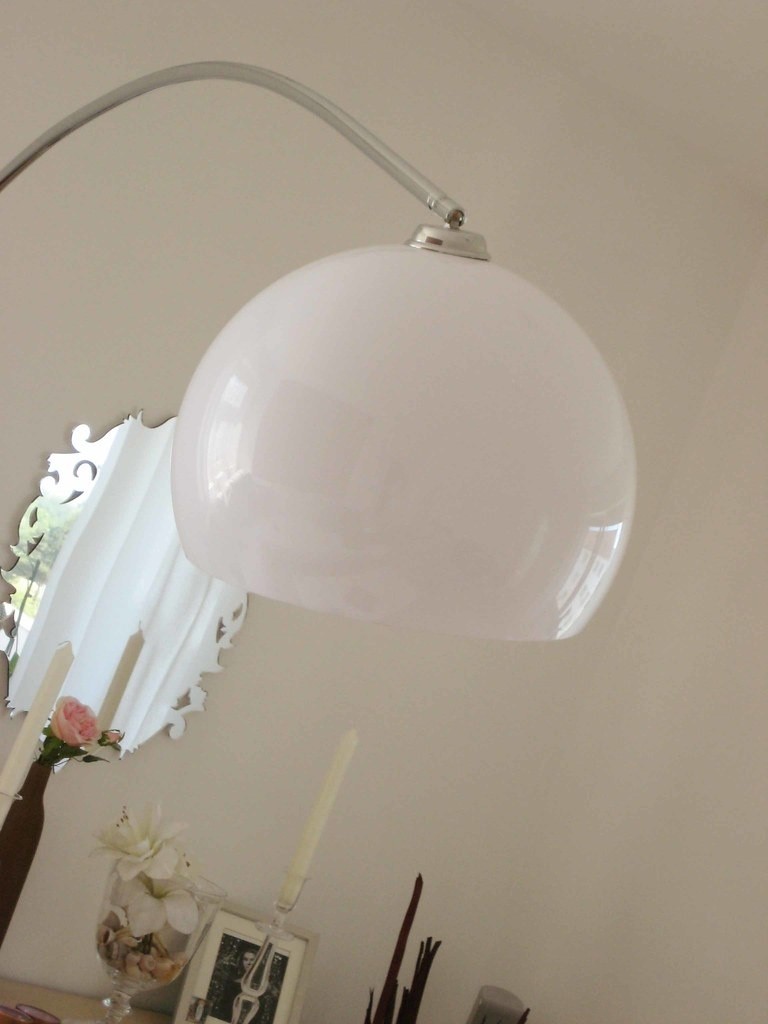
[92,857,229,1024]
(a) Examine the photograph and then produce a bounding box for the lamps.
[0,61,638,645]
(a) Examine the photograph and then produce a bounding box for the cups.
[0,1003,63,1024]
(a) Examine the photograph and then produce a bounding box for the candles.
[86,620,145,749]
[276,721,360,911]
[0,640,75,831]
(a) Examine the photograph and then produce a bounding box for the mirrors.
[0,408,250,773]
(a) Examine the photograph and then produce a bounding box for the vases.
[0,761,51,950]
[95,860,228,1024]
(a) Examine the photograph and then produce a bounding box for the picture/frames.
[173,901,321,1024]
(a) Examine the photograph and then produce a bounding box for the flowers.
[88,800,207,952]
[35,696,126,764]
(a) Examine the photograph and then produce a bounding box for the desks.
[0,979,174,1024]
